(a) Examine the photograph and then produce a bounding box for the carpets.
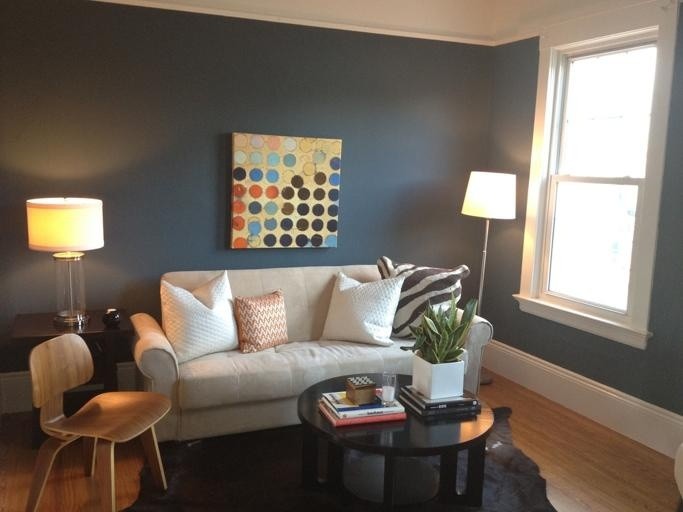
[123,407,557,512]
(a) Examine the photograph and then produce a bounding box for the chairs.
[24,331,173,512]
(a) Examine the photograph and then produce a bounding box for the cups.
[380,373,397,406]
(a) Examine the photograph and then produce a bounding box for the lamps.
[460,170,518,386]
[22,192,108,326]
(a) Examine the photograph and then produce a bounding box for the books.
[319,385,481,427]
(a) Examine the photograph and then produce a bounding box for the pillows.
[230,289,290,354]
[153,267,240,364]
[316,270,408,347]
[377,255,472,340]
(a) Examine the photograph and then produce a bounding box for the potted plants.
[402,288,480,400]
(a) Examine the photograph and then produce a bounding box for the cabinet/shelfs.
[9,308,133,448]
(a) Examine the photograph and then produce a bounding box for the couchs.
[131,257,492,445]
